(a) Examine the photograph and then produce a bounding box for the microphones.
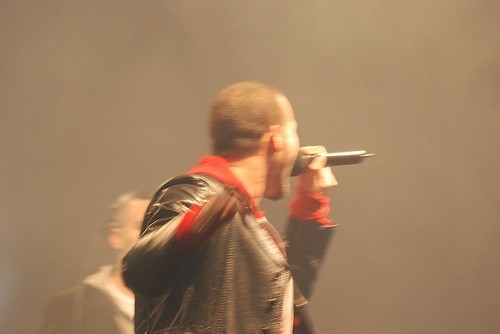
[290,150,375,177]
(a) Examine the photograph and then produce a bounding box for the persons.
[48,194,154,333]
[120,83,339,332]
